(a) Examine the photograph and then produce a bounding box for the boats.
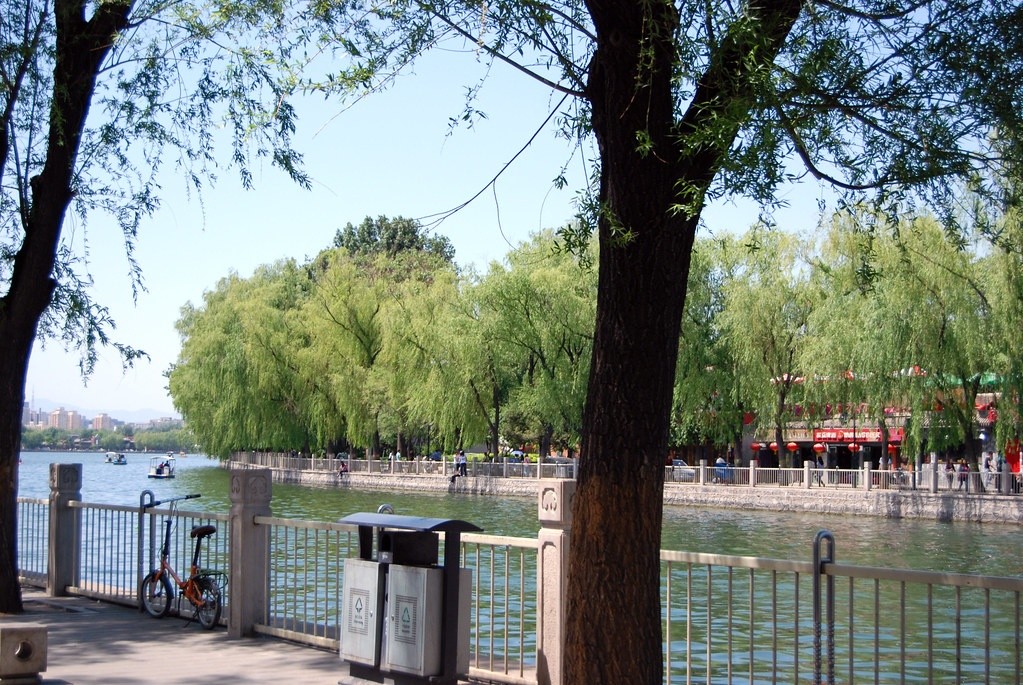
[105,452,116,464]
[180,451,187,457]
[147,456,176,478]
[113,454,127,464]
[164,450,176,460]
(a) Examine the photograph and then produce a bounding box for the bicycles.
[138,493,228,629]
[712,471,735,484]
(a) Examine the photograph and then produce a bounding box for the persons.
[816,456,825,487]
[396,450,401,461]
[159,461,170,468]
[945,458,969,491]
[716,454,725,463]
[388,450,393,460]
[337,462,347,479]
[454,450,467,476]
[513,454,531,463]
[984,450,1004,488]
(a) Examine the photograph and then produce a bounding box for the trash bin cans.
[339,512,484,685]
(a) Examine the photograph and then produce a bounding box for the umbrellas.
[512,451,524,454]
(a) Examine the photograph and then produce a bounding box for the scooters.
[890,469,910,485]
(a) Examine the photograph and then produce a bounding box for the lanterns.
[751,443,761,450]
[787,442,798,451]
[887,444,892,453]
[743,413,751,424]
[770,442,779,455]
[848,443,860,452]
[813,443,824,453]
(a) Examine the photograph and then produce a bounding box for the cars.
[675,460,695,482]
[531,457,578,477]
[484,459,522,476]
[335,452,362,471]
